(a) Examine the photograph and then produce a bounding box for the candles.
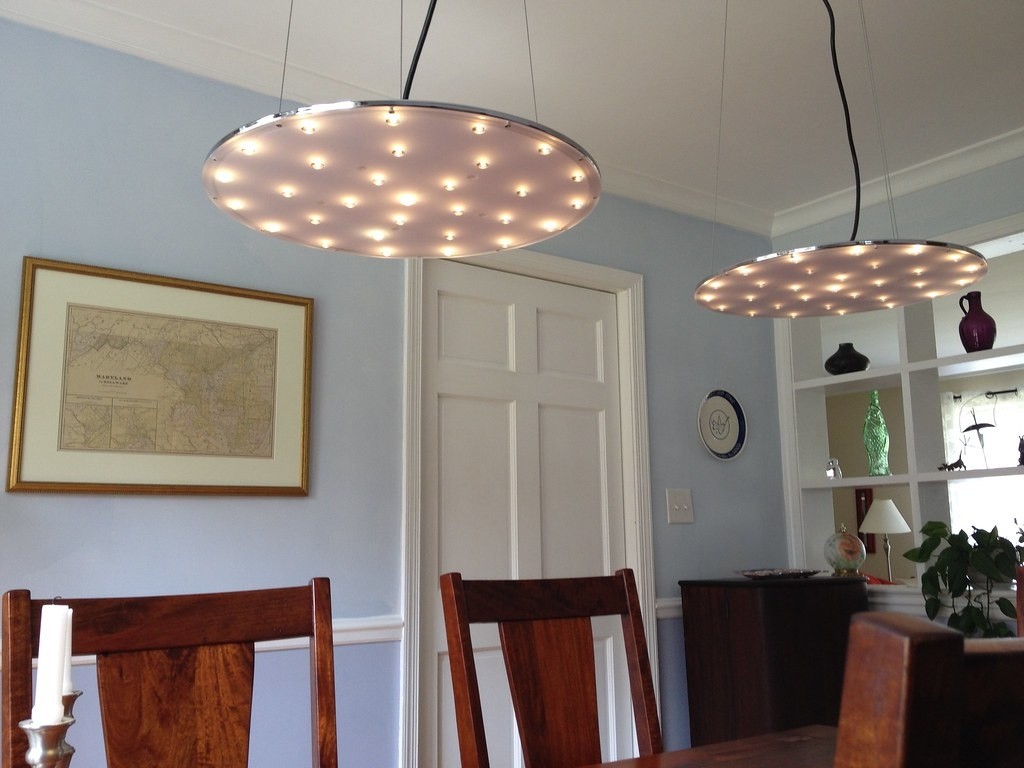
[30,595,73,725]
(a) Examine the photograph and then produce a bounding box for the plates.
[740,568,820,580]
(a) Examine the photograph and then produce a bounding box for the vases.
[958,291,996,352]
[825,342,871,374]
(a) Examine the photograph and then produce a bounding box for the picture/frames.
[6,254,314,499]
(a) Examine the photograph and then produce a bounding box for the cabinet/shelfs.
[680,576,868,746]
[771,126,1023,631]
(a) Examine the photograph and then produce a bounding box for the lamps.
[859,499,910,581]
[693,1,986,316]
[199,1,601,258]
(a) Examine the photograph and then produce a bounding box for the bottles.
[959,291,996,353]
[825,459,842,479]
[825,343,870,375]
[863,388,889,475]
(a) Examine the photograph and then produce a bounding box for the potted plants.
[903,520,1024,639]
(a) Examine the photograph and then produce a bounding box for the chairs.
[440,570,666,768]
[2,575,338,768]
